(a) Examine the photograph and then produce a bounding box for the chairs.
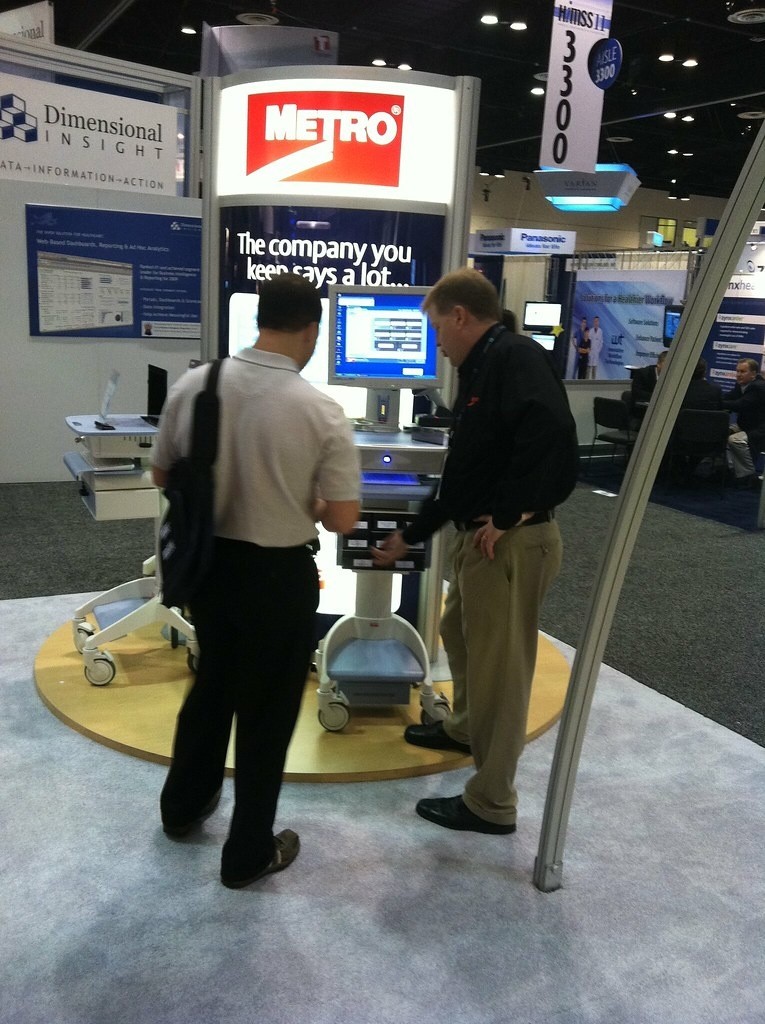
[588,396,639,473]
[668,408,729,489]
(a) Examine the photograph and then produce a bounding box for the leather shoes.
[417,795,516,835]
[405,720,471,754]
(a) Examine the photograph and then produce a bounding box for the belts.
[454,509,556,531]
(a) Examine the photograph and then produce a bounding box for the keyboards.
[80,449,135,471]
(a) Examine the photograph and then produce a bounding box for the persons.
[148,273,361,890]
[368,265,581,836]
[632,351,689,476]
[725,358,765,490]
[589,315,603,380]
[683,356,728,481]
[577,326,591,379]
[571,317,587,379]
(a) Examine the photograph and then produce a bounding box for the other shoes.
[731,476,747,485]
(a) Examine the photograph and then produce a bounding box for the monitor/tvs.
[521,302,561,335]
[146,364,168,416]
[663,304,683,348]
[329,283,446,423]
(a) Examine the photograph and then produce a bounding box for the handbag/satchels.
[159,359,220,606]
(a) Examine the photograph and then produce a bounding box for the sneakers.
[222,829,301,890]
[163,786,222,835]
